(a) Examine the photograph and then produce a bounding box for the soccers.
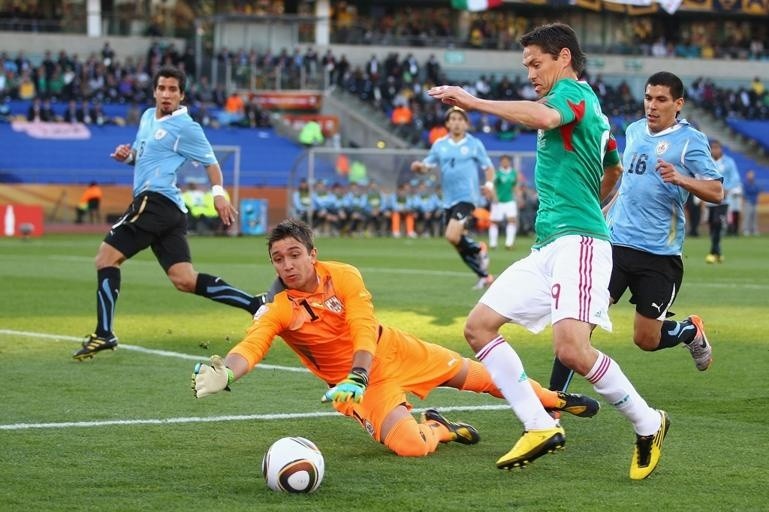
[261,435,326,495]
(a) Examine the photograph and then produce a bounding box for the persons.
[408,105,498,289]
[0,0,528,249]
[515,0,769,264]
[187,217,601,459]
[65,65,271,360]
[543,68,727,419]
[427,21,673,482]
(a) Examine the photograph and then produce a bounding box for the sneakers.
[496,419,568,471]
[541,387,599,418]
[479,240,488,271]
[471,274,493,289]
[255,295,270,324]
[682,316,711,370]
[421,411,479,445]
[73,334,116,361]
[630,410,670,480]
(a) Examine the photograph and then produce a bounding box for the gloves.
[191,355,233,399]
[320,367,369,402]
